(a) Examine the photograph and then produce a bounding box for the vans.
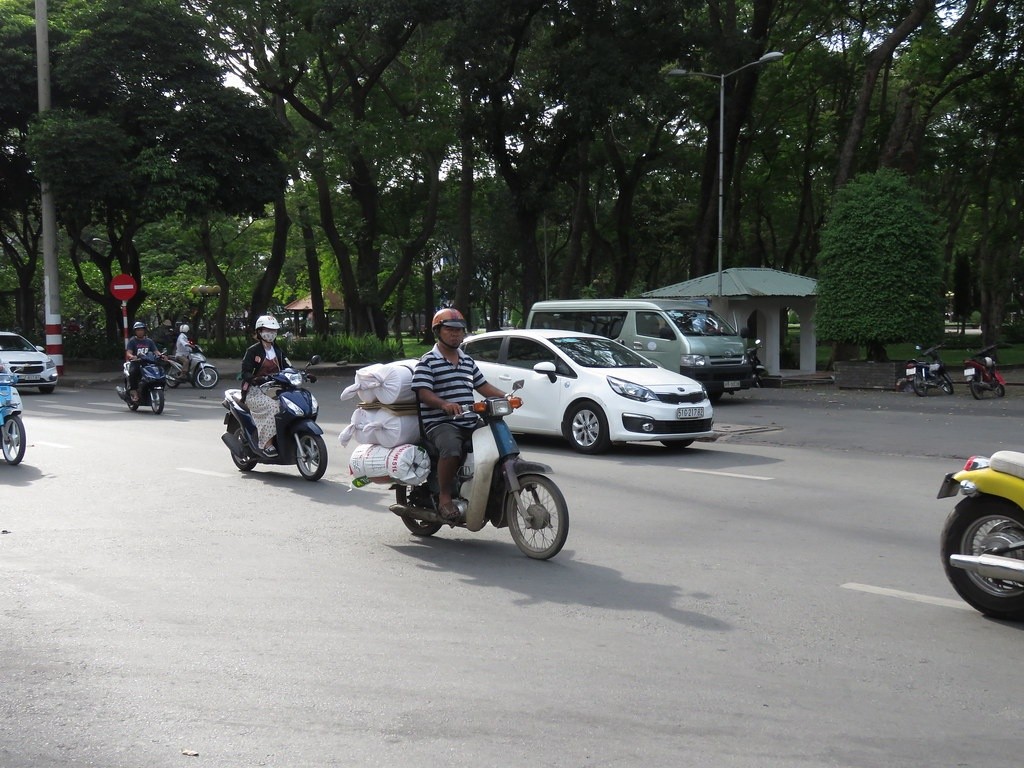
[524,297,755,405]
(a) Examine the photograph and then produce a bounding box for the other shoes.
[180,374,187,380]
[131,395,139,402]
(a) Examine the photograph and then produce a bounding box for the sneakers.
[262,446,279,458]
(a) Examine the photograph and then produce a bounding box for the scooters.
[220,355,328,482]
[744,338,767,389]
[0,363,30,466]
[116,348,168,414]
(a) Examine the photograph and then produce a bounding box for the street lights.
[663,52,786,296]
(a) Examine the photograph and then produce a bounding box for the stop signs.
[111,274,137,300]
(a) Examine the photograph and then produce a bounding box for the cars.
[456,328,715,454]
[0,331,58,395]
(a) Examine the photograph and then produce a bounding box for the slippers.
[438,501,460,520]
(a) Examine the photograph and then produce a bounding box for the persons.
[125,322,168,402]
[175,324,195,380]
[240,315,317,457]
[153,319,173,354]
[285,329,293,345]
[410,309,521,521]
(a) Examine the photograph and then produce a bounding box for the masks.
[260,332,278,343]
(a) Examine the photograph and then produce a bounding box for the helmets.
[432,308,467,328]
[255,315,280,330]
[180,324,190,333]
[133,322,146,330]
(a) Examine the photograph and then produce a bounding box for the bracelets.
[504,394,509,398]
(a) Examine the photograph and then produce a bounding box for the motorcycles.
[162,339,219,388]
[962,340,1007,400]
[935,449,1024,621]
[387,378,570,560]
[905,342,954,396]
